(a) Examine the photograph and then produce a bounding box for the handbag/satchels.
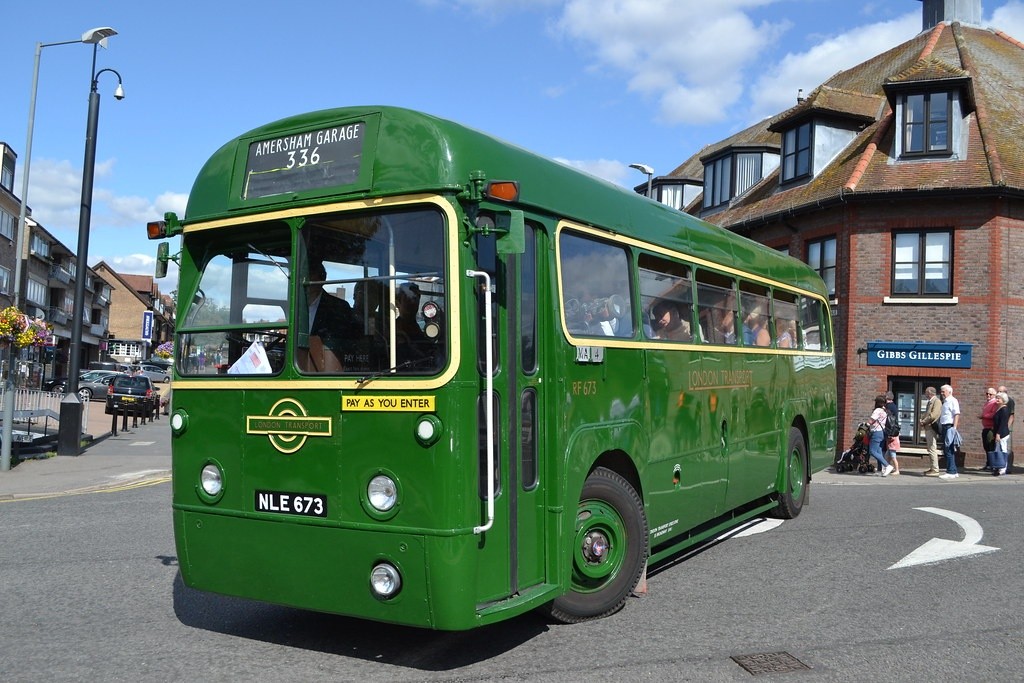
[988,441,1008,468]
[932,417,943,434]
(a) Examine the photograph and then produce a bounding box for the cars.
[45,362,171,401]
[104,373,161,417]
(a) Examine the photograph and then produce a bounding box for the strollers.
[836,422,874,474]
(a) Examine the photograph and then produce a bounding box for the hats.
[875,396,885,402]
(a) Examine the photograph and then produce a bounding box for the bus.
[145,102,841,635]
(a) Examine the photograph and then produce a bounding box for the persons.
[939,384,961,480]
[350,276,379,371]
[978,385,1015,476]
[214,350,222,374]
[564,279,801,348]
[395,282,427,371]
[921,387,942,477]
[284,261,354,371]
[837,391,900,477]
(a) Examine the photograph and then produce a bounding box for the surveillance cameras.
[114,87,125,100]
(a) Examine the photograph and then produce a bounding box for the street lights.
[54,63,126,458]
[628,164,654,199]
[1,24,121,474]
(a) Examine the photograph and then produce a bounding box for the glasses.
[986,392,993,396]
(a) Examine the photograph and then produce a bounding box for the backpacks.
[876,407,901,437]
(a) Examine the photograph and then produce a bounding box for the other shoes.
[875,471,884,477]
[980,465,992,472]
[921,469,932,476]
[926,470,939,477]
[837,460,845,463]
[992,468,998,474]
[994,472,1001,476]
[939,473,955,479]
[1006,469,1011,474]
[891,471,900,475]
[882,465,894,477]
[955,473,959,478]
